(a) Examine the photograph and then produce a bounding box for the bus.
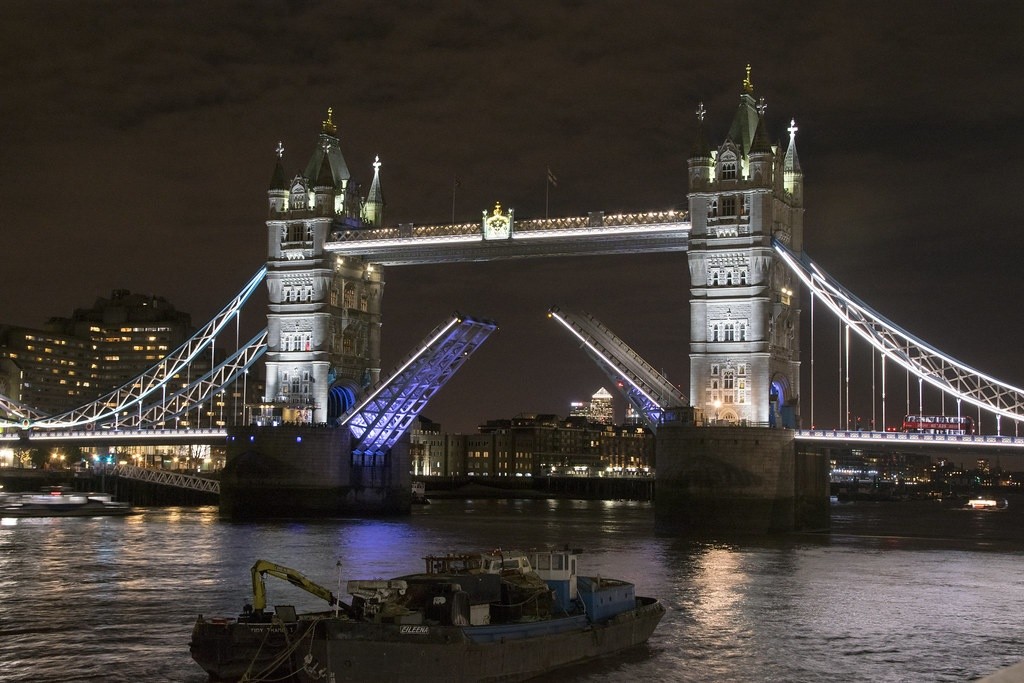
[903,414,977,435]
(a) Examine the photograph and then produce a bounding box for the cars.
[888,424,902,432]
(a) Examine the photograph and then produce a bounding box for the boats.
[0,486,133,518]
[188,541,666,683]
[836,475,1024,501]
